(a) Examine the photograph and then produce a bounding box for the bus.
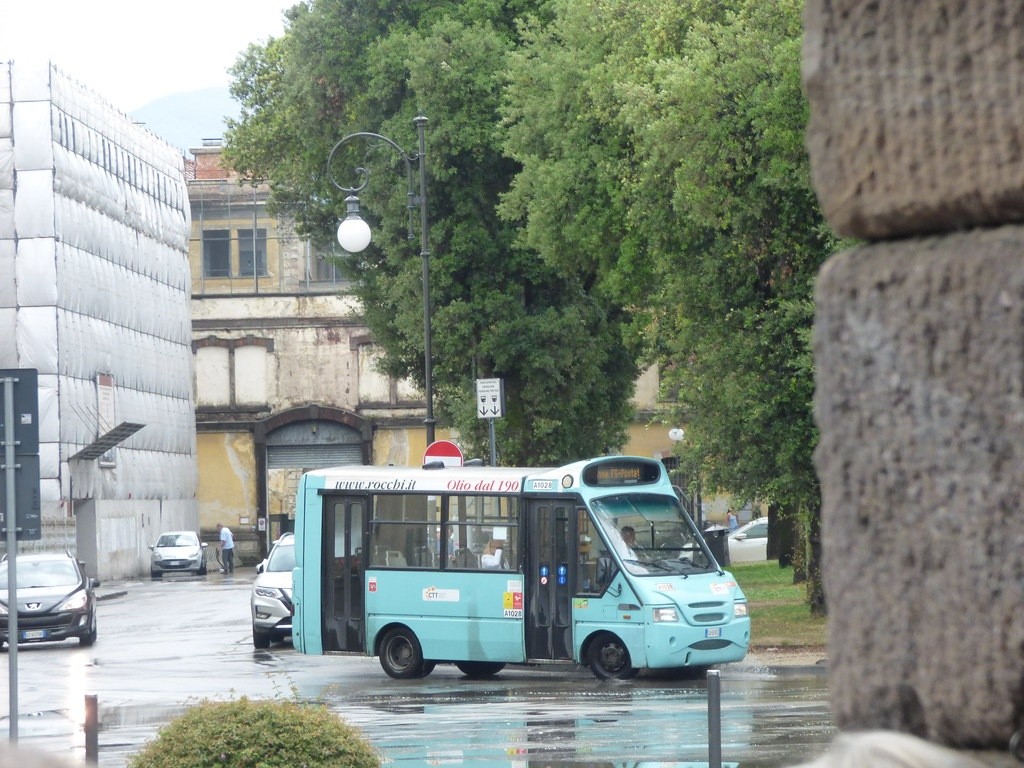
[289,455,751,682]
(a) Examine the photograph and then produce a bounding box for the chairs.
[355,545,478,568]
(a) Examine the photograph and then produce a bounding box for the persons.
[480,539,504,570]
[620,526,649,562]
[725,507,737,529]
[216,523,235,575]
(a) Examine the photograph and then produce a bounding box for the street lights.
[670,428,683,458]
[326,133,438,449]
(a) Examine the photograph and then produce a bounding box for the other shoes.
[220,570,229,574]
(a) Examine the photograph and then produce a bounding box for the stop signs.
[423,440,464,468]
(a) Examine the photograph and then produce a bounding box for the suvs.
[250,531,294,649]
[1,549,101,650]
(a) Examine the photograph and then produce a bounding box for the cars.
[148,531,208,577]
[679,516,769,567]
